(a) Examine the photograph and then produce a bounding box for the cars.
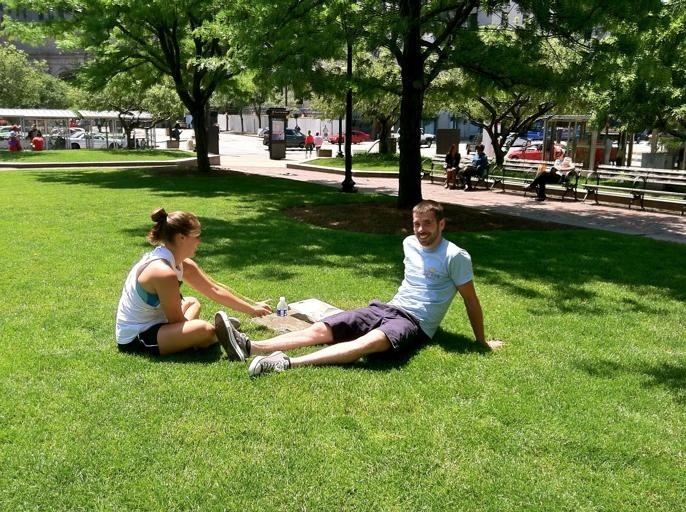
[327,130,370,145]
[503,133,528,147]
[397,127,436,149]
[466,134,482,154]
[48,127,122,149]
[258,126,315,151]
[508,141,573,161]
[0,125,32,139]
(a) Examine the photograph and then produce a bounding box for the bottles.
[276,296,288,333]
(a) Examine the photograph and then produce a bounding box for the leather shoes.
[523,188,531,191]
[535,198,544,201]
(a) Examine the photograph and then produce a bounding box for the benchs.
[423,153,686,216]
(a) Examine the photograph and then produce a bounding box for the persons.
[186,136,196,150]
[522,151,575,201]
[295,125,301,132]
[323,125,328,139]
[8,124,65,151]
[172,123,183,141]
[445,144,487,191]
[304,130,323,157]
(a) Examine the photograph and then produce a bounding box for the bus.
[526,115,589,146]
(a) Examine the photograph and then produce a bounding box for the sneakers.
[249,350,290,376]
[444,184,472,191]
[214,311,249,361]
